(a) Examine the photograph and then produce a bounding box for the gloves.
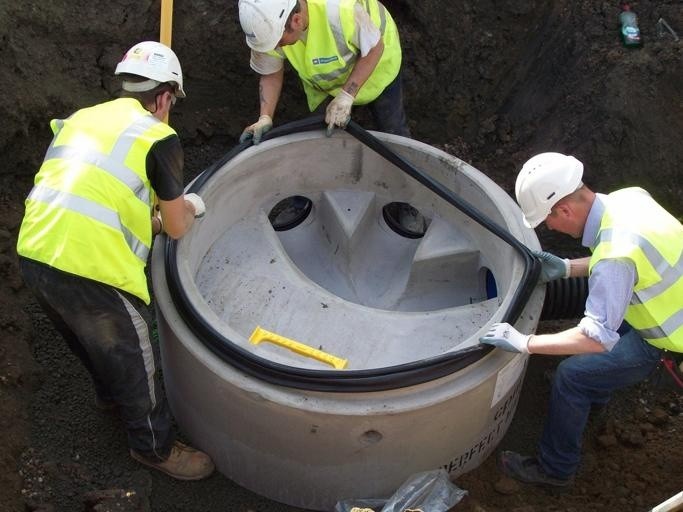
[239,114,274,146]
[156,192,207,234]
[326,89,355,136]
[479,322,534,354]
[530,250,571,286]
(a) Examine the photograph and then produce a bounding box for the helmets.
[515,151,584,229]
[114,41,186,99]
[238,1,299,54]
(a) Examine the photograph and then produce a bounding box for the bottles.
[618,4,642,48]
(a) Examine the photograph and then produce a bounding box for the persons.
[16,40,216,481]
[236,0,412,145]
[479,152,683,488]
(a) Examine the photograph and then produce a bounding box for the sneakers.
[500,450,574,491]
[92,396,119,410]
[130,439,216,482]
